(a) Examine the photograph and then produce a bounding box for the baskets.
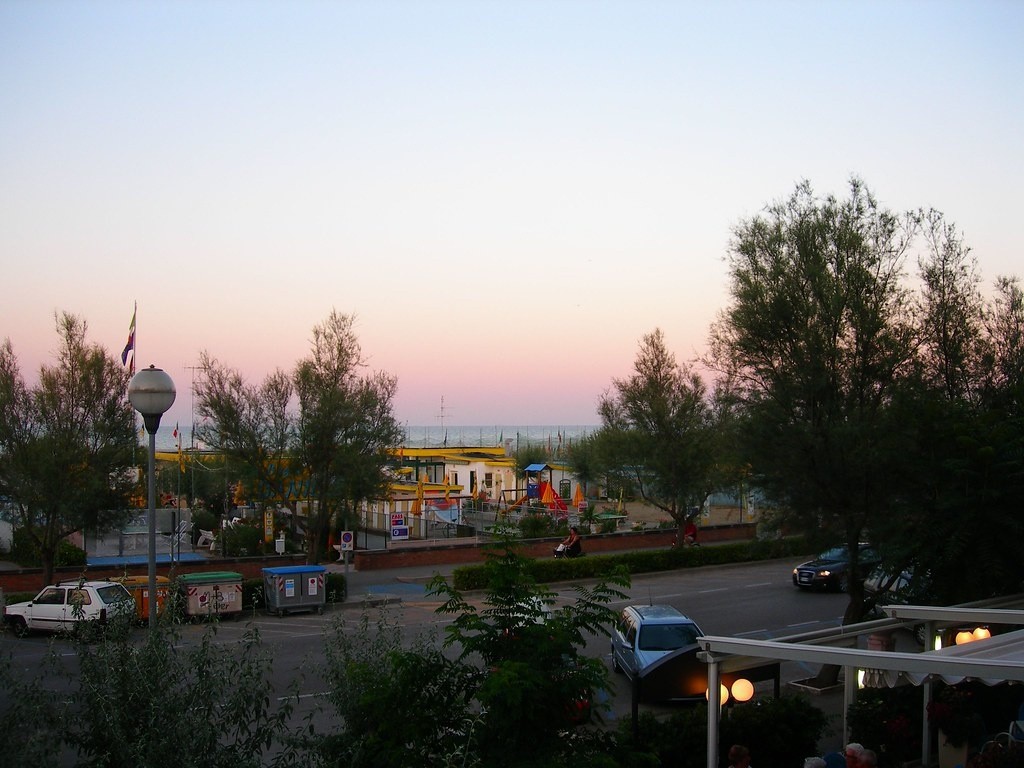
[554,550,563,559]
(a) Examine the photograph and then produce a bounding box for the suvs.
[3,578,135,645]
[610,605,706,702]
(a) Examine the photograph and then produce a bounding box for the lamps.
[956,622,991,644]
[706,678,754,716]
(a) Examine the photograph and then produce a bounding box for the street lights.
[128,364,176,634]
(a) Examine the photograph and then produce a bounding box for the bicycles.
[554,545,586,560]
[671,535,701,550]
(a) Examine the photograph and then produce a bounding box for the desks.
[119,525,149,549]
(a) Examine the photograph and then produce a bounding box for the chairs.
[159,518,194,547]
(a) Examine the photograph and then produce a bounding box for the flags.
[472,477,479,500]
[177,441,185,474]
[173,429,177,438]
[416,472,424,505]
[558,431,561,443]
[121,312,136,381]
[140,424,144,439]
[445,475,451,503]
[443,433,447,446]
[500,431,504,442]
[400,447,403,457]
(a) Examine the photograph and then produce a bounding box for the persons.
[684,514,700,547]
[481,480,487,493]
[728,745,753,768]
[495,480,505,498]
[563,527,582,558]
[804,742,877,768]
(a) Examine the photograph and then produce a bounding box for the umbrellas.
[542,480,555,510]
[573,483,585,513]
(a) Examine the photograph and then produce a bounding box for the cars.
[793,540,932,605]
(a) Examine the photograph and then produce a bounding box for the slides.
[500,493,533,513]
